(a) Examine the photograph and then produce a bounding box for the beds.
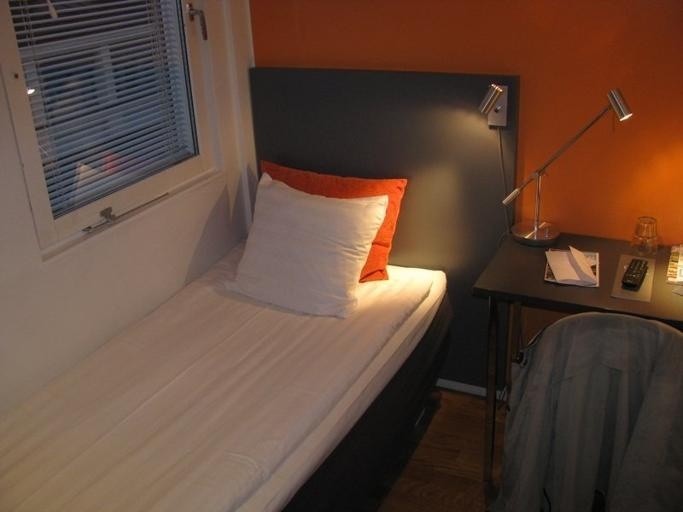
[1,238,454,512]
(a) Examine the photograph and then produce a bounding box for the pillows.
[223,172,390,318]
[258,159,407,283]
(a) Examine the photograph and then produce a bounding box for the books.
[544,247,601,288]
[667,244,683,285]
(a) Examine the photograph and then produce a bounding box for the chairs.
[513,311,683,511]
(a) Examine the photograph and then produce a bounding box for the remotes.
[621,259,649,290]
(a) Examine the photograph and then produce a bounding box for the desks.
[471,232,683,512]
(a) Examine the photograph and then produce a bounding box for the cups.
[631,216,658,256]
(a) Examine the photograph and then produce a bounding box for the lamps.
[501,86,635,249]
[478,83,510,130]
